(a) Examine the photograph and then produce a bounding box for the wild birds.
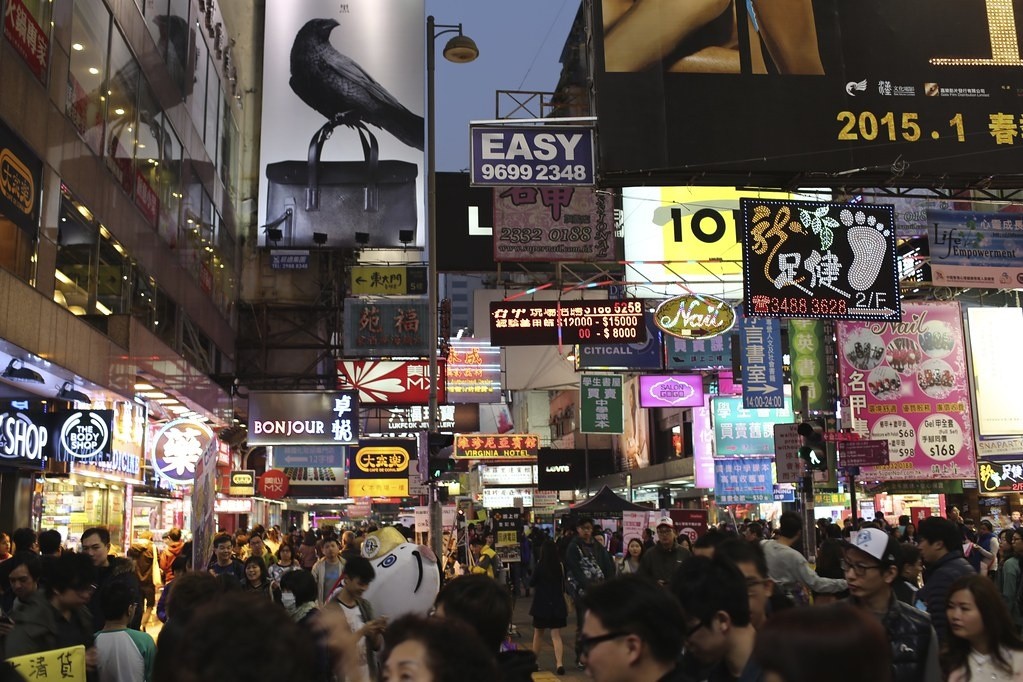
[288,18,424,152]
[43,15,200,146]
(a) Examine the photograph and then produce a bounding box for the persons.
[0,506,1023,682]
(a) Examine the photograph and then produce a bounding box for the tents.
[569,485,654,516]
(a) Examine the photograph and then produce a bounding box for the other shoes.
[531,663,539,672]
[556,666,566,675]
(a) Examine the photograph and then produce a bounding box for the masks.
[282,592,295,608]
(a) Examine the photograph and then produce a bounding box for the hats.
[656,516,674,528]
[841,527,906,574]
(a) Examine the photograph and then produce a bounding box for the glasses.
[575,630,643,658]
[657,530,673,536]
[747,577,771,596]
[1012,537,1022,541]
[998,538,1006,542]
[578,527,591,531]
[838,556,880,579]
[677,621,707,640]
